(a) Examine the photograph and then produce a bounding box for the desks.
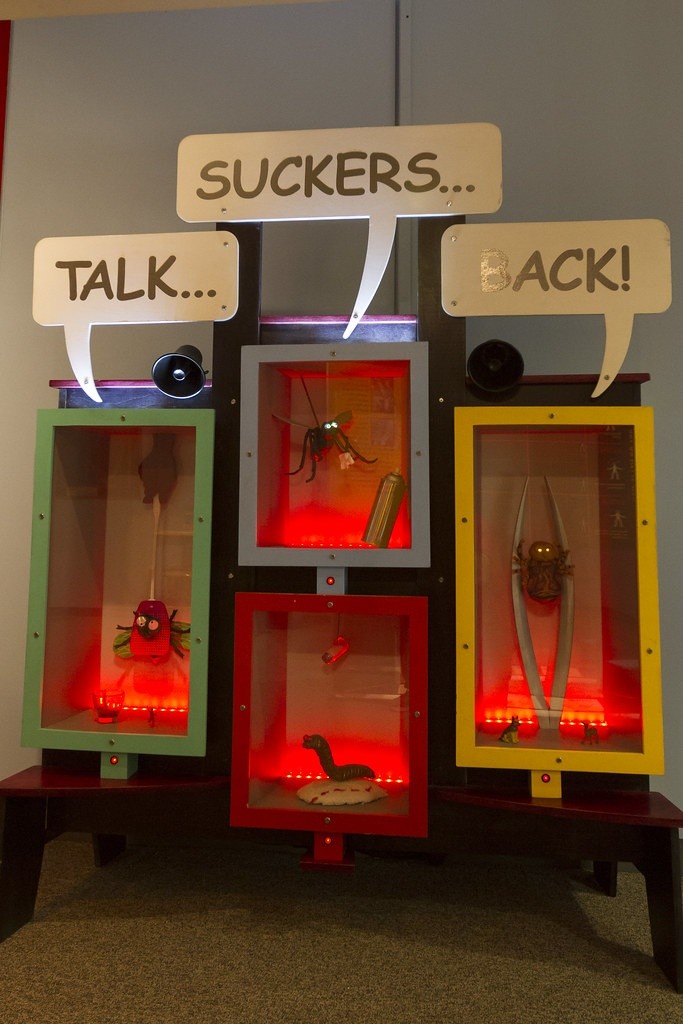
[1,770,683,994]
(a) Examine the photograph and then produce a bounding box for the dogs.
[581,721,599,745]
[499,715,521,744]
